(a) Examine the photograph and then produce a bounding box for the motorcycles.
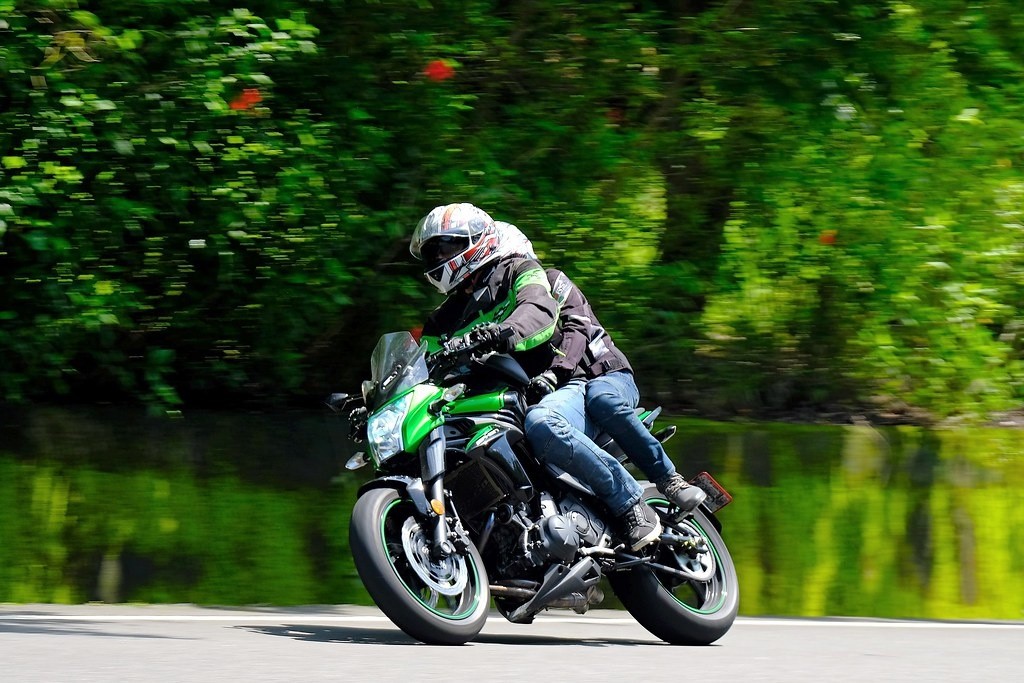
[322,329,742,646]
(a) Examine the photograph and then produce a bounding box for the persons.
[349,203,707,552]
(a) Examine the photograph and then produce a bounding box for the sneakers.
[664,474,707,513]
[622,497,661,552]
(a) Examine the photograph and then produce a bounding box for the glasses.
[419,236,469,267]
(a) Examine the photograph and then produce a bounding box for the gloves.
[527,370,563,398]
[469,322,502,358]
[346,406,368,430]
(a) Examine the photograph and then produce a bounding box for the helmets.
[409,203,500,295]
[494,221,537,261]
